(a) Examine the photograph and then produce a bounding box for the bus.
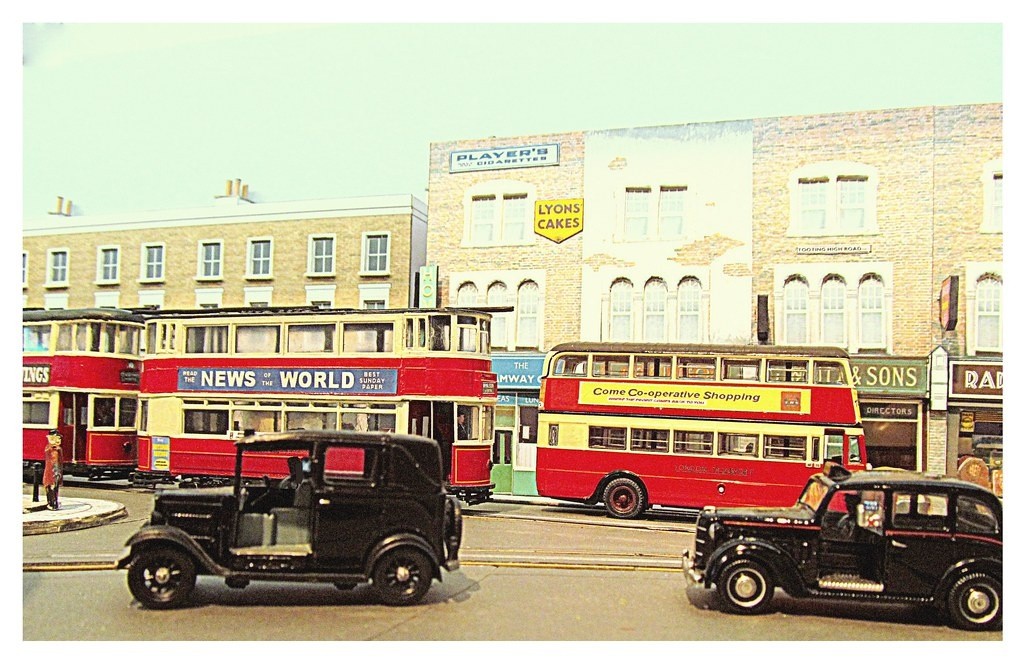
[136,352,499,508]
[535,341,868,519]
[23,351,142,479]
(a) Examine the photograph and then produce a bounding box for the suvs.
[113,428,463,607]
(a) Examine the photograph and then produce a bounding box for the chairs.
[270,456,313,544]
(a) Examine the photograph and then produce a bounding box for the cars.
[680,462,1003,632]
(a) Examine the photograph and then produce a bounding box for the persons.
[42,430,63,511]
[446,412,467,480]
